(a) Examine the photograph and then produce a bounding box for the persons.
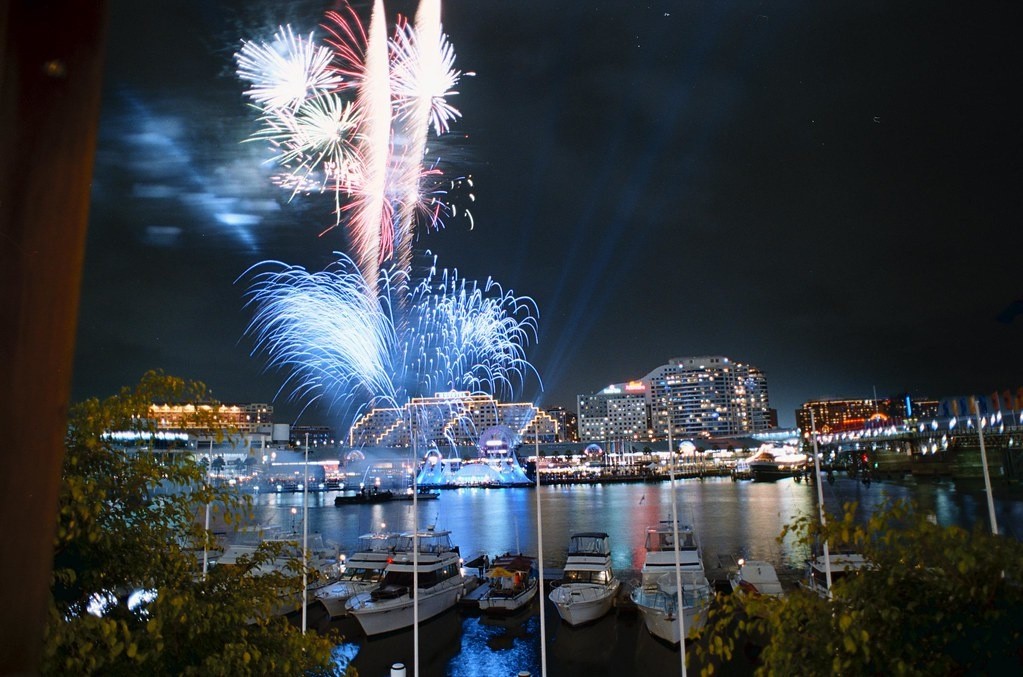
[478,552,529,598]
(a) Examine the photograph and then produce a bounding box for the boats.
[477,561,539,617]
[548,529,623,630]
[183,458,442,631]
[721,535,787,614]
[795,541,886,618]
[727,451,805,483]
[312,513,410,623]
[345,511,481,643]
[628,490,717,653]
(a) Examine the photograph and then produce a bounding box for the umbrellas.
[485,567,514,578]
